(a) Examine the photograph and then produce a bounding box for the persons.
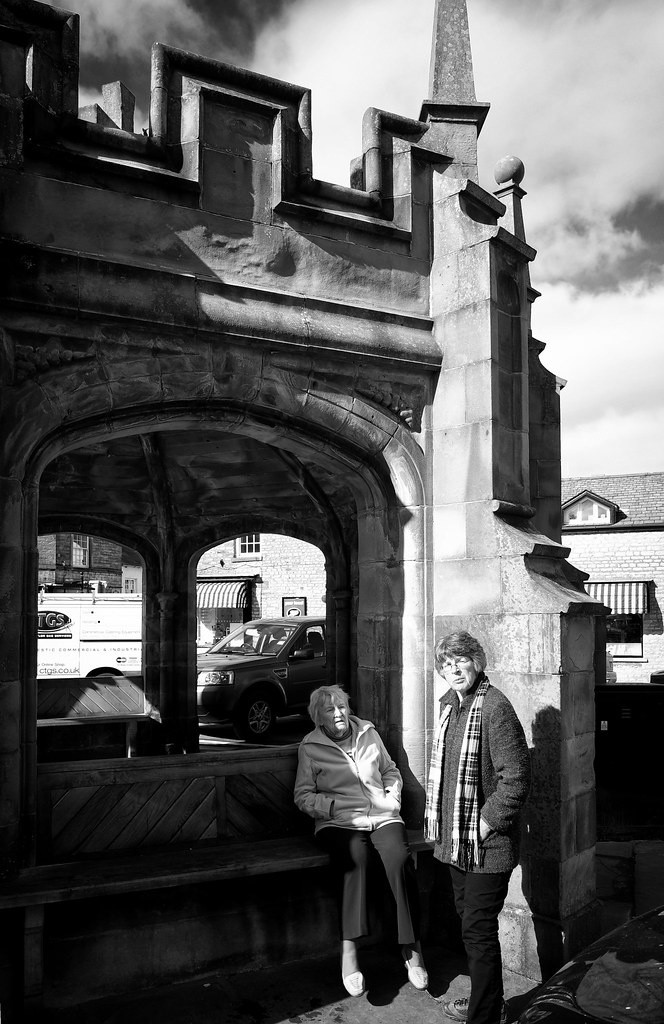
[421,632,531,1024]
[295,685,428,997]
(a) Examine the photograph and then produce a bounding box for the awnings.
[196,583,247,609]
[584,583,649,614]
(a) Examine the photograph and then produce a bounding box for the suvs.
[195,616,331,742]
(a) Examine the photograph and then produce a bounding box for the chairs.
[307,632,324,652]
[262,628,287,654]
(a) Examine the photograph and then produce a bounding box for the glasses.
[439,655,471,677]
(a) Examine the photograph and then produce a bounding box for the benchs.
[0,827,437,1024]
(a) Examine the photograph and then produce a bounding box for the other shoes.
[401,945,429,990]
[338,940,365,998]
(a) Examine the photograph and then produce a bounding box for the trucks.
[36,589,142,677]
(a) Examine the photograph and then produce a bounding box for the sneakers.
[442,996,509,1023]
